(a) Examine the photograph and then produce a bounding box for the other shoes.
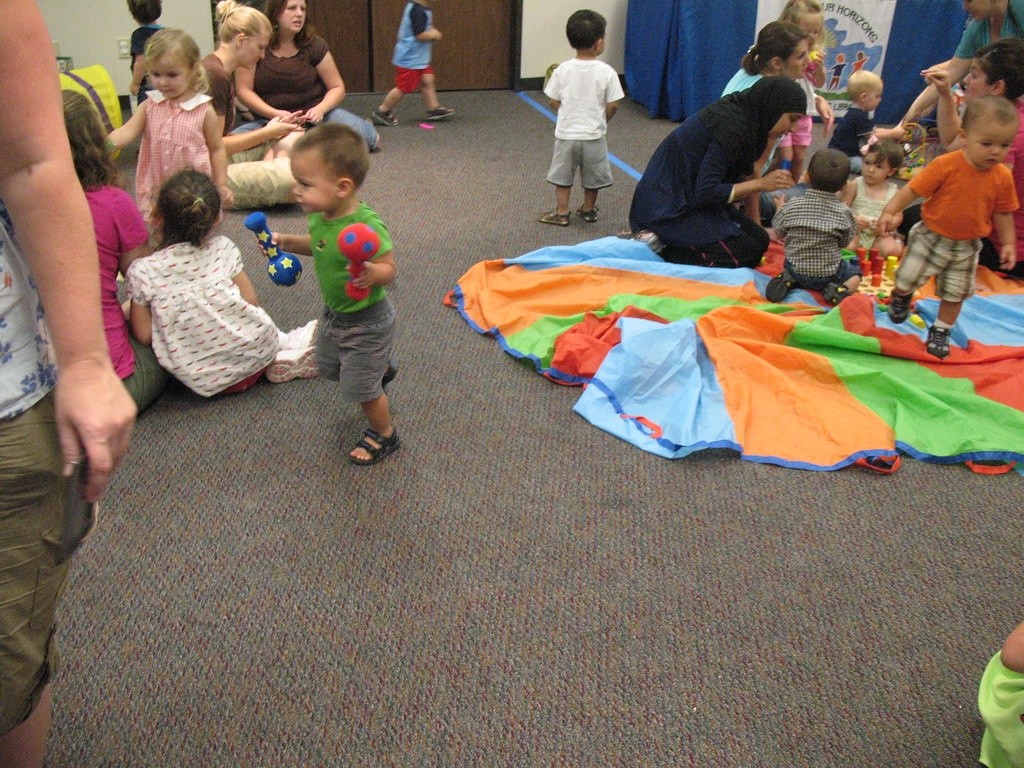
[576,203,599,221]
[266,345,322,383]
[821,280,851,306]
[616,228,662,253]
[536,206,571,225]
[765,267,796,303]
[282,318,320,351]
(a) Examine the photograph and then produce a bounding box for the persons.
[824,71,907,156]
[103,28,237,244]
[255,123,400,467]
[838,137,904,258]
[370,0,456,127]
[0,0,138,768]
[978,621,1024,768]
[875,96,1019,360]
[764,149,863,306]
[614,0,833,269]
[62,88,178,415]
[119,168,321,398]
[125,0,167,108]
[871,0,1024,279]
[537,9,627,225]
[199,0,381,208]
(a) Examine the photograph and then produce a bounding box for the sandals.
[370,106,400,127]
[349,428,400,466]
[426,106,456,121]
[381,358,397,388]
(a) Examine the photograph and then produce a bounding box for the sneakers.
[926,325,950,359]
[888,290,913,324]
